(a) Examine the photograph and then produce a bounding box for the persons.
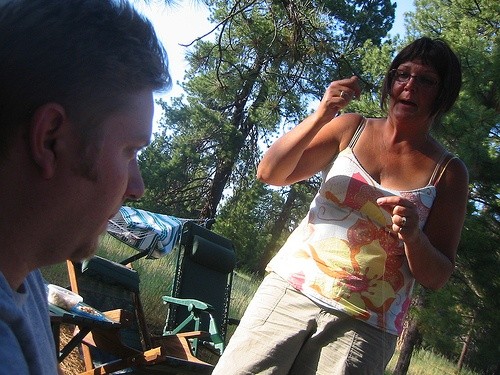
[0,1,171,375]
[210,36,470,375]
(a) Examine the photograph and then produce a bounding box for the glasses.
[387,68,436,88]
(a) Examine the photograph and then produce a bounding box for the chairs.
[65,251,215,375]
[159,220,242,362]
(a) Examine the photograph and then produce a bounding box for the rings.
[397,226,405,233]
[340,90,345,97]
[401,217,406,226]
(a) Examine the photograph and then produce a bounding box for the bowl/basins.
[47,283,83,312]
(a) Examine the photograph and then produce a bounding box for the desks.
[44,283,124,363]
